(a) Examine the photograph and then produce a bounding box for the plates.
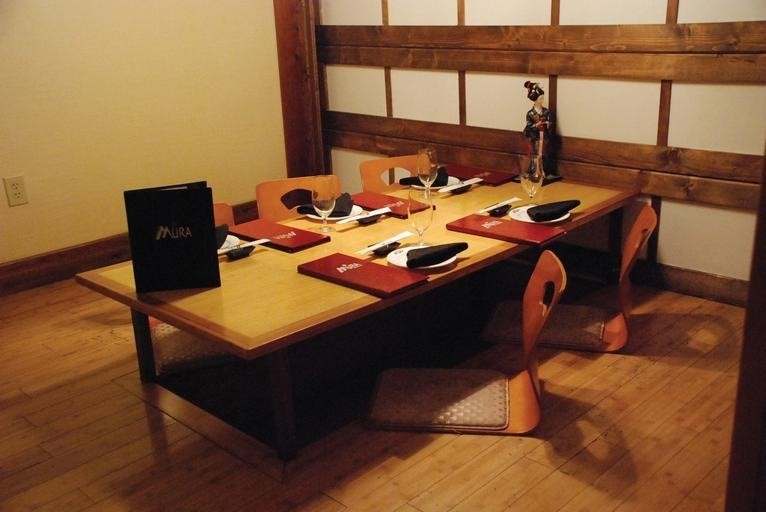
[305,205,363,221]
[485,204,511,216]
[356,214,382,226]
[217,234,240,252]
[451,184,471,195]
[369,241,401,256]
[386,246,455,271]
[509,204,569,225]
[226,243,255,261]
[410,176,459,189]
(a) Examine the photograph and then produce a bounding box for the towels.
[526,197,582,222]
[397,163,448,188]
[212,222,230,250]
[402,238,472,271]
[295,191,355,219]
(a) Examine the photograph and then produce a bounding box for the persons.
[520,80,561,180]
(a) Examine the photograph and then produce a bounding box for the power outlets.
[2,173,29,208]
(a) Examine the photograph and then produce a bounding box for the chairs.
[481,197,661,356]
[349,243,572,442]
[146,198,248,379]
[252,173,345,223]
[358,151,432,196]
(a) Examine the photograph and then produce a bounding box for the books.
[346,189,430,220]
[224,217,331,254]
[296,252,431,299]
[446,213,568,250]
[443,165,519,186]
[123,180,222,294]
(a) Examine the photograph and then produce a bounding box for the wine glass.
[416,147,438,210]
[407,198,433,245]
[311,189,337,232]
[519,154,545,205]
